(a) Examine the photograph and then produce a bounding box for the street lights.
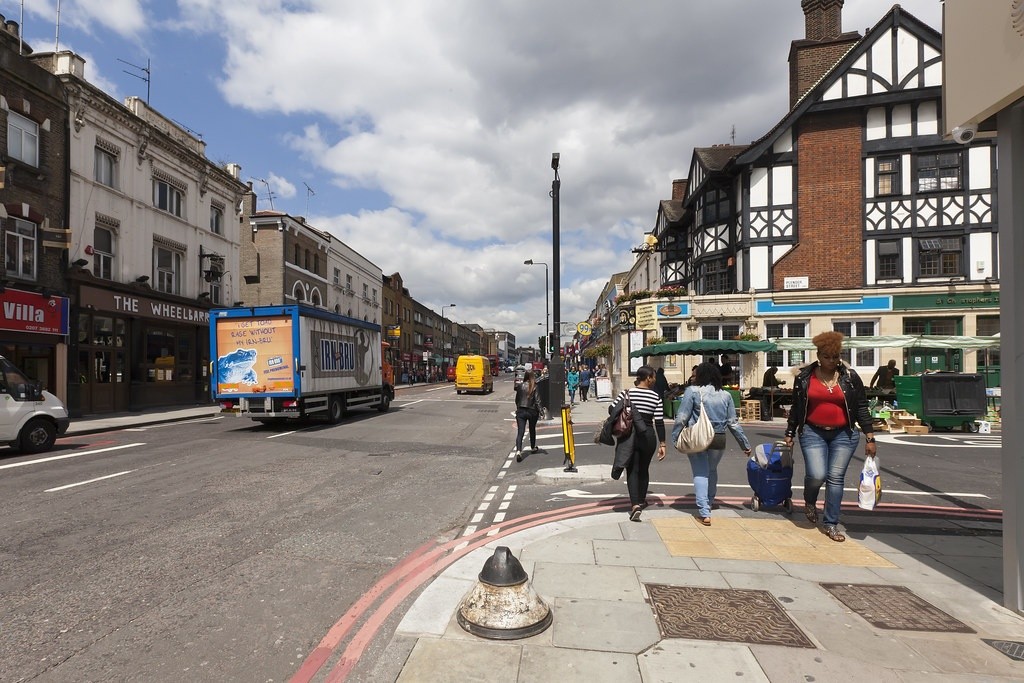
[441,304,458,382]
[525,260,550,363]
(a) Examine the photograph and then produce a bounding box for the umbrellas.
[629,338,778,385]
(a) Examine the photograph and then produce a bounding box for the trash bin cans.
[893,370,988,432]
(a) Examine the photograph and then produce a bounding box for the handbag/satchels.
[675,385,715,453]
[857,454,881,511]
[612,388,634,439]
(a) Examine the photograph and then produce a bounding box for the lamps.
[234,301,244,305]
[73,259,88,267]
[199,292,209,297]
[135,274,149,282]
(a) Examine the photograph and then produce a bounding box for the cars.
[2,355,78,453]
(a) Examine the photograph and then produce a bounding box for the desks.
[752,392,793,421]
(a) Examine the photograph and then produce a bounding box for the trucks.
[208,302,396,424]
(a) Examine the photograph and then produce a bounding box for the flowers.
[612,286,687,302]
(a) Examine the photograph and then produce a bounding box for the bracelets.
[659,445,666,447]
[785,430,796,438]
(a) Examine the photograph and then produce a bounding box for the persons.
[607,365,666,521]
[671,362,751,527]
[762,366,786,387]
[406,368,425,387]
[514,369,545,462]
[784,330,877,543]
[565,363,606,406]
[870,359,900,390]
[652,353,733,411]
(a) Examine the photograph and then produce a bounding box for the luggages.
[747,440,794,514]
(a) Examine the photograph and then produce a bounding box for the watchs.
[865,438,875,443]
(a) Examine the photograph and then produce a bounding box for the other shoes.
[630,500,648,520]
[517,454,521,462]
[804,503,818,524]
[824,524,845,542]
[530,446,538,453]
[698,516,711,525]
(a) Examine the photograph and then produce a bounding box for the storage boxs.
[975,420,990,434]
[985,387,1001,397]
[857,406,929,435]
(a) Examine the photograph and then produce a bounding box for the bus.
[485,354,498,377]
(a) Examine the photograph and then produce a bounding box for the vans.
[456,353,494,394]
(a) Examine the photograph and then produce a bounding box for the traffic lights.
[549,331,554,353]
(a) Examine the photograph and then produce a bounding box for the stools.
[741,400,760,423]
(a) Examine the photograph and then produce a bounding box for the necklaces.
[818,366,838,394]
